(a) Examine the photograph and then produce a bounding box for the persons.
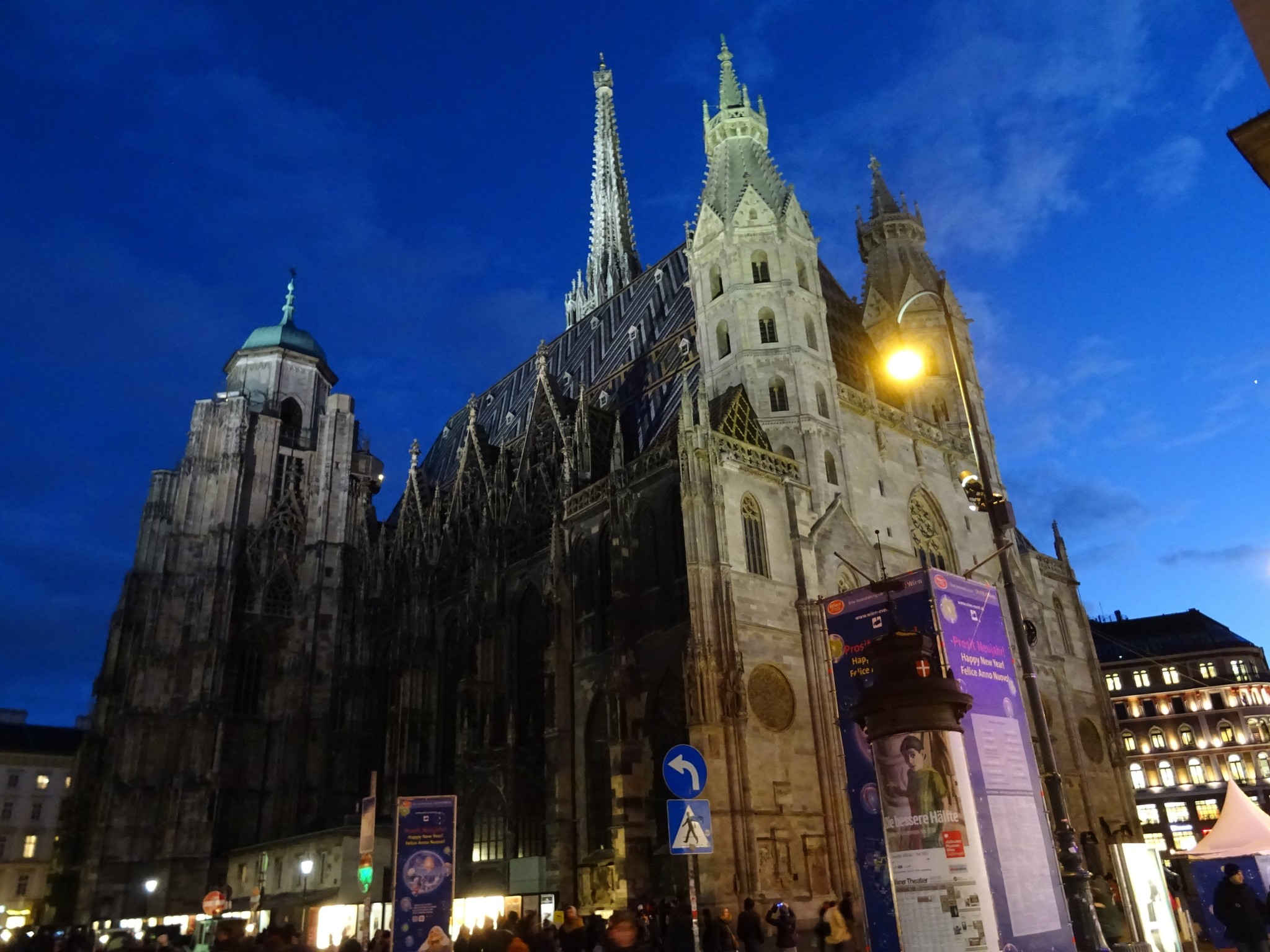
[0,889,864,952]
[1210,862,1270,952]
[1088,871,1130,944]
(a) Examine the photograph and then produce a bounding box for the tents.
[1189,776,1270,947]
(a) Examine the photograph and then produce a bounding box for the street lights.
[299,852,315,952]
[143,874,160,944]
[884,288,1114,952]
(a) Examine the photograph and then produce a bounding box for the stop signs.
[202,889,225,919]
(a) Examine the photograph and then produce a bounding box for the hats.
[824,893,838,901]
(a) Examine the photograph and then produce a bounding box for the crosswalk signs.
[667,799,715,855]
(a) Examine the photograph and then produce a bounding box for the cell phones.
[777,903,783,906]
